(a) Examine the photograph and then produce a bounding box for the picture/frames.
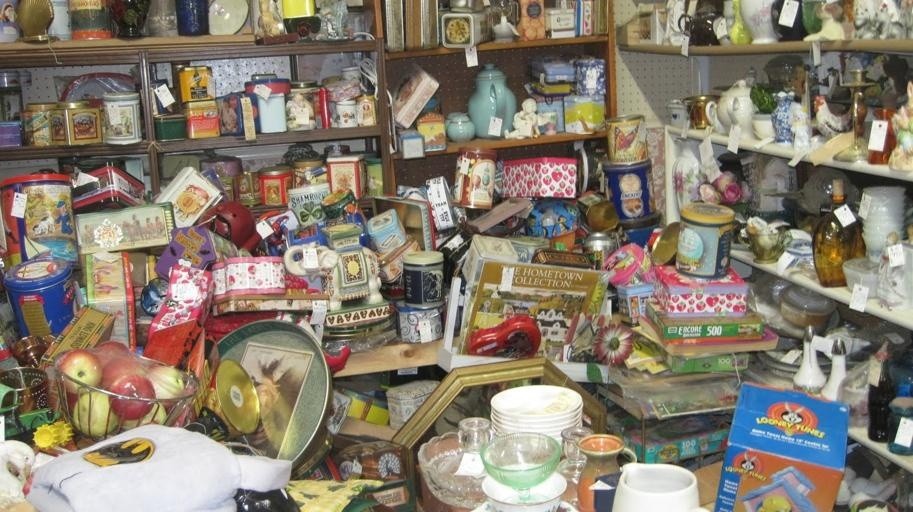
[389,356,611,512]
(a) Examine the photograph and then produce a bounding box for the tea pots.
[717,79,756,142]
[678,5,719,45]
[704,99,726,136]
[468,63,518,138]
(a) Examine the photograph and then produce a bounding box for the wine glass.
[478,431,561,505]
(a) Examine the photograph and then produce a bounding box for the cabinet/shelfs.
[661,119,911,511]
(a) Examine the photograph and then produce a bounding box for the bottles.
[340,67,361,83]
[542,108,558,135]
[895,469,909,512]
[792,324,827,396]
[366,157,383,197]
[866,352,895,443]
[810,177,866,289]
[821,339,848,402]
[357,89,378,128]
[885,397,912,457]
[867,94,898,165]
[335,99,358,129]
[729,1,751,45]
[555,425,593,507]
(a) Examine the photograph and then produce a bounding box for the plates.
[757,350,831,366]
[755,353,831,373]
[760,368,792,379]
[488,384,583,433]
[207,0,248,35]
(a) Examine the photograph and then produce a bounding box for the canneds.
[3,255,77,337]
[676,200,736,280]
[603,166,658,220]
[452,145,495,209]
[606,113,648,165]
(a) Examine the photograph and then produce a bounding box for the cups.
[609,462,710,512]
[146,0,176,37]
[736,227,793,265]
[107,1,149,38]
[834,467,856,506]
[458,417,490,453]
[859,184,906,265]
[176,1,207,35]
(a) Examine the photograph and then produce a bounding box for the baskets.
[51,349,199,441]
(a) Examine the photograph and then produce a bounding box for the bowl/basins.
[479,465,567,512]
[415,431,486,511]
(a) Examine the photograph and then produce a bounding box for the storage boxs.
[452,233,607,362]
[649,261,752,319]
[715,384,850,511]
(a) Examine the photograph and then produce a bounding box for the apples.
[51,342,182,442]
